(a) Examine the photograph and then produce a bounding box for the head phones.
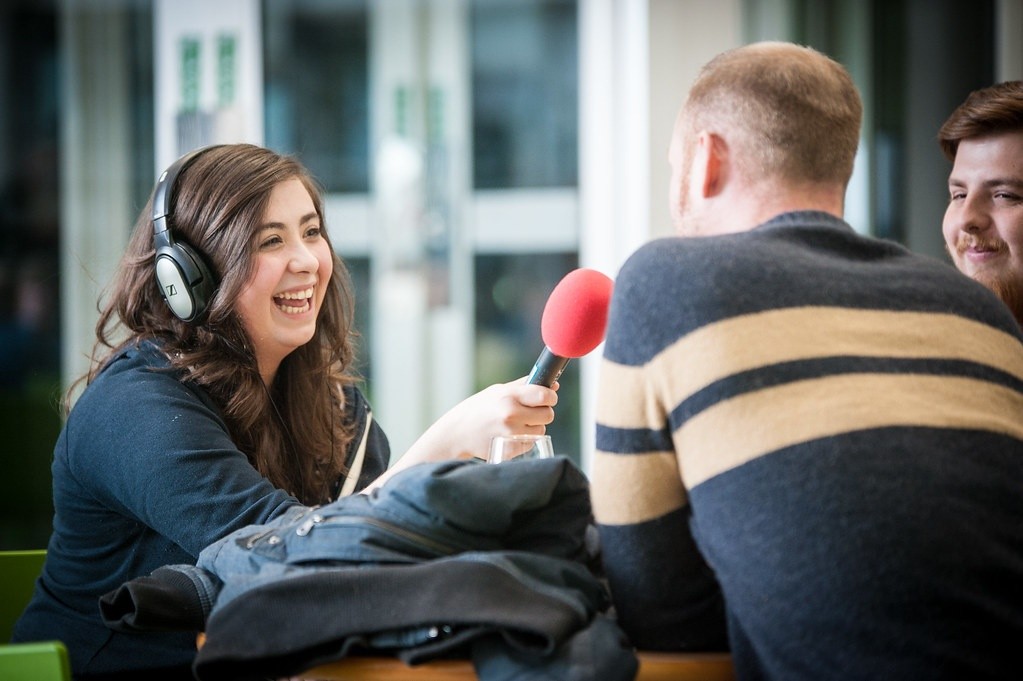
[154,144,215,325]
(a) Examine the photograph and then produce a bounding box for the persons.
[588,38,1021,681]
[15,143,560,681]
[940,81,1022,319]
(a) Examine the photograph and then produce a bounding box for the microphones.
[528,268,616,388]
